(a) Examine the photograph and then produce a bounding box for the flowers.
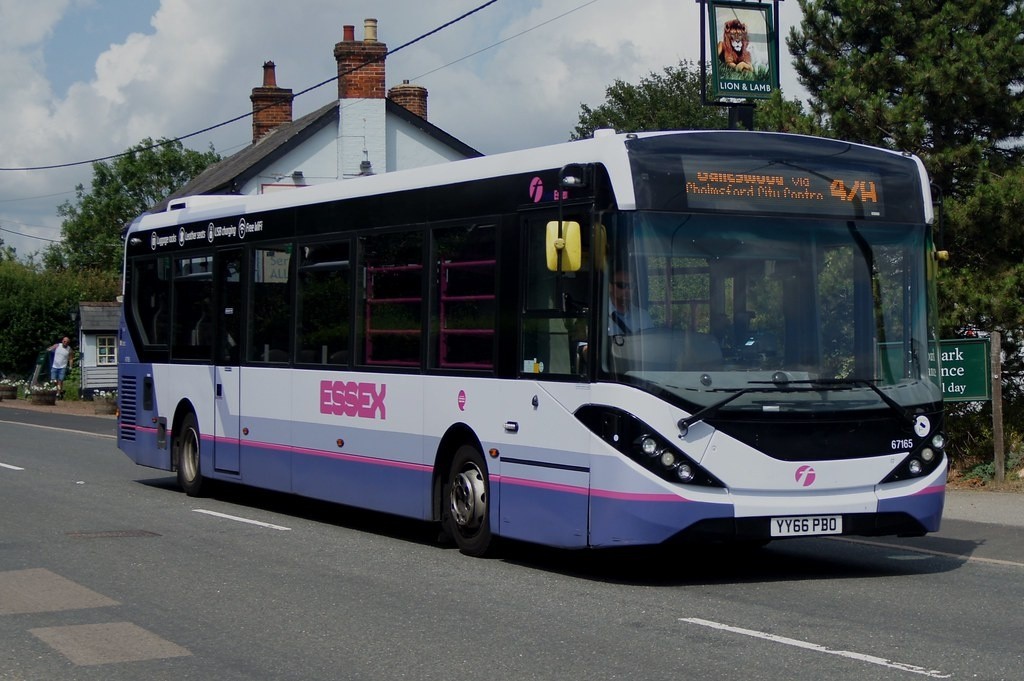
[0,378,25,387]
[28,379,61,392]
[94,388,119,398]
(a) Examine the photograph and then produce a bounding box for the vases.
[94,395,118,414]
[31,389,59,405]
[0,385,19,399]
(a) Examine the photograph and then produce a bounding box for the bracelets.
[69,367,72,369]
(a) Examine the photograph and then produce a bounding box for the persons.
[47,337,74,400]
[577,270,655,362]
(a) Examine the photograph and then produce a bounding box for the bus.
[113,121,956,569]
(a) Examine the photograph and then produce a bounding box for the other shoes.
[56,393,61,399]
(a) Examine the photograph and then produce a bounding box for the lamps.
[361,160,370,169]
[291,170,304,178]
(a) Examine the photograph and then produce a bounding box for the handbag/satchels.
[49,343,59,369]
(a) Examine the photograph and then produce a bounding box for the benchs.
[144,270,349,365]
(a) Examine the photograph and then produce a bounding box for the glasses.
[610,281,635,290]
